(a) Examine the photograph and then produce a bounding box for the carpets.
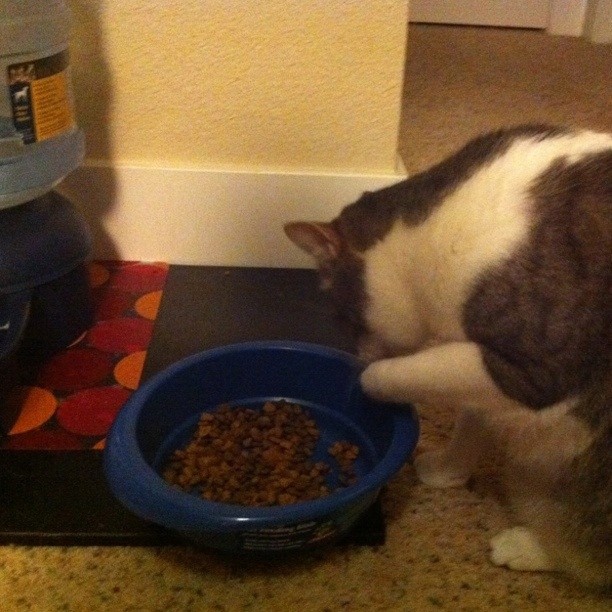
[2,256,386,549]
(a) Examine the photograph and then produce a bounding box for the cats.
[282,119,612,591]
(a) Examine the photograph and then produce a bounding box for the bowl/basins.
[104,337,421,556]
